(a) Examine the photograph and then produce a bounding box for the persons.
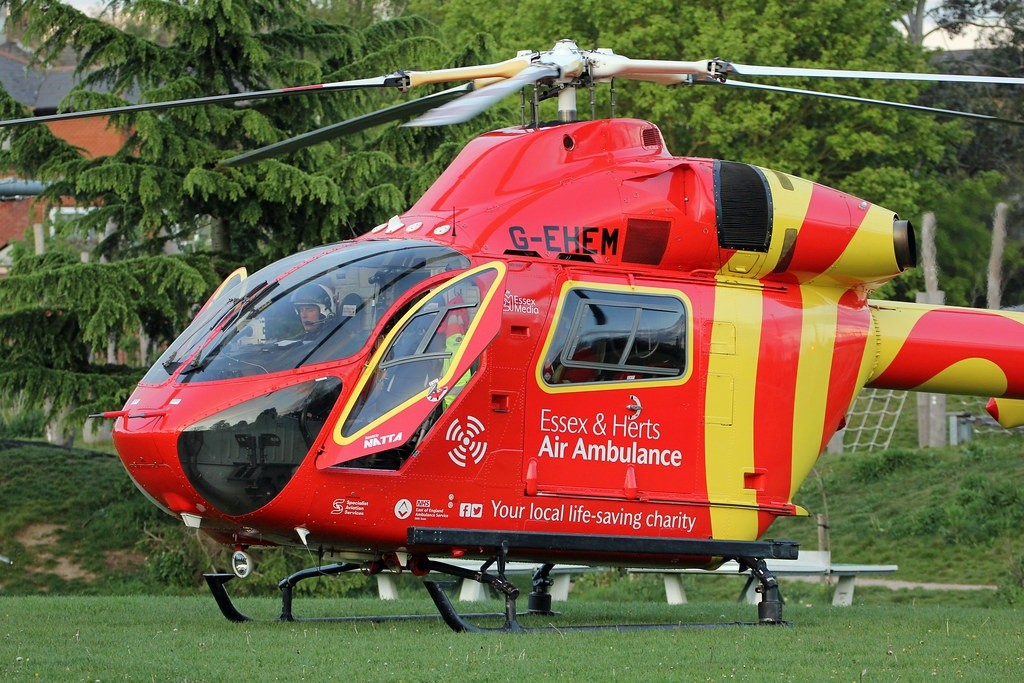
[291,281,346,374]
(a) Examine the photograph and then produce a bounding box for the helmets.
[290,282,337,321]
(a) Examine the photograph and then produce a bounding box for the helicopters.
[0,38,1024,629]
